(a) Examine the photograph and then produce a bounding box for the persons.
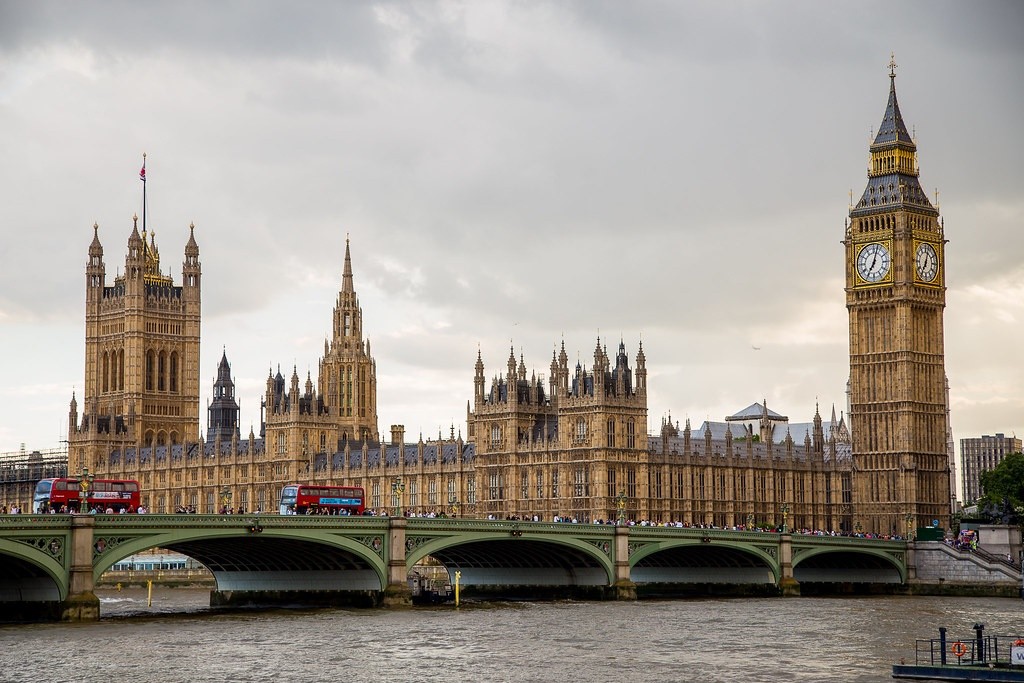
[944,535,963,551]
[1008,552,1011,564]
[486,511,917,544]
[0,503,458,523]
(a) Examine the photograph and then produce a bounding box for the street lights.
[747,512,755,531]
[75,466,94,514]
[614,489,628,528]
[951,492,957,524]
[906,508,914,543]
[780,501,789,534]
[392,477,406,518]
[448,496,460,519]
[219,485,232,514]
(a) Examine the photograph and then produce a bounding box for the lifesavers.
[951,642,966,656]
[1012,638,1024,647]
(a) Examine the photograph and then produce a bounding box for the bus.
[32,477,141,514]
[280,484,364,515]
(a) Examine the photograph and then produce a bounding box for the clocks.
[858,244,890,281]
[916,243,938,281]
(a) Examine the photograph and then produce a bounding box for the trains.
[105,553,205,571]
